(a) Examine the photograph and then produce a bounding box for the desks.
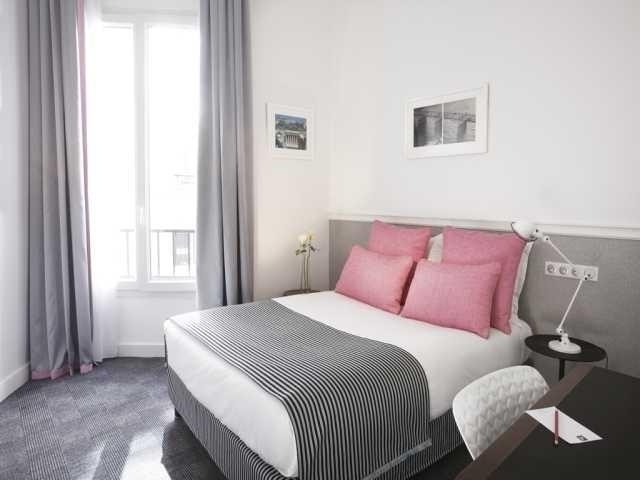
[453,363,640,480]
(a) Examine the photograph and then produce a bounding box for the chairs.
[450,361,550,463]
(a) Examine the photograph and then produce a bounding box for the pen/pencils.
[554,408,558,445]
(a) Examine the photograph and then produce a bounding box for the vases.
[297,254,312,291]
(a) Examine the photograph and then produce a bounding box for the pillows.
[332,215,536,342]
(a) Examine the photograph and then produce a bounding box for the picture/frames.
[403,83,490,160]
[266,100,313,159]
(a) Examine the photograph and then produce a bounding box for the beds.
[160,287,534,480]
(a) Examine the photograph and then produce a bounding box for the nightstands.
[283,288,320,296]
[522,331,609,383]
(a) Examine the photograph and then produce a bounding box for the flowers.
[294,229,319,289]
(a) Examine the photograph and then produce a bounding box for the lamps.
[507,211,598,356]
